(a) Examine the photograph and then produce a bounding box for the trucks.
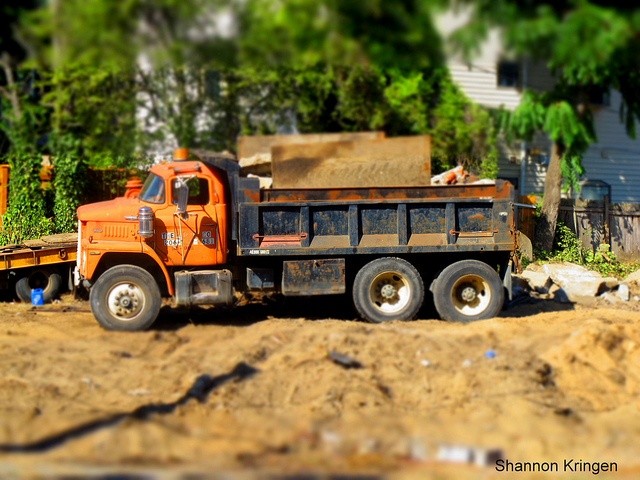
[0,164,144,303]
[73,162,515,330]
